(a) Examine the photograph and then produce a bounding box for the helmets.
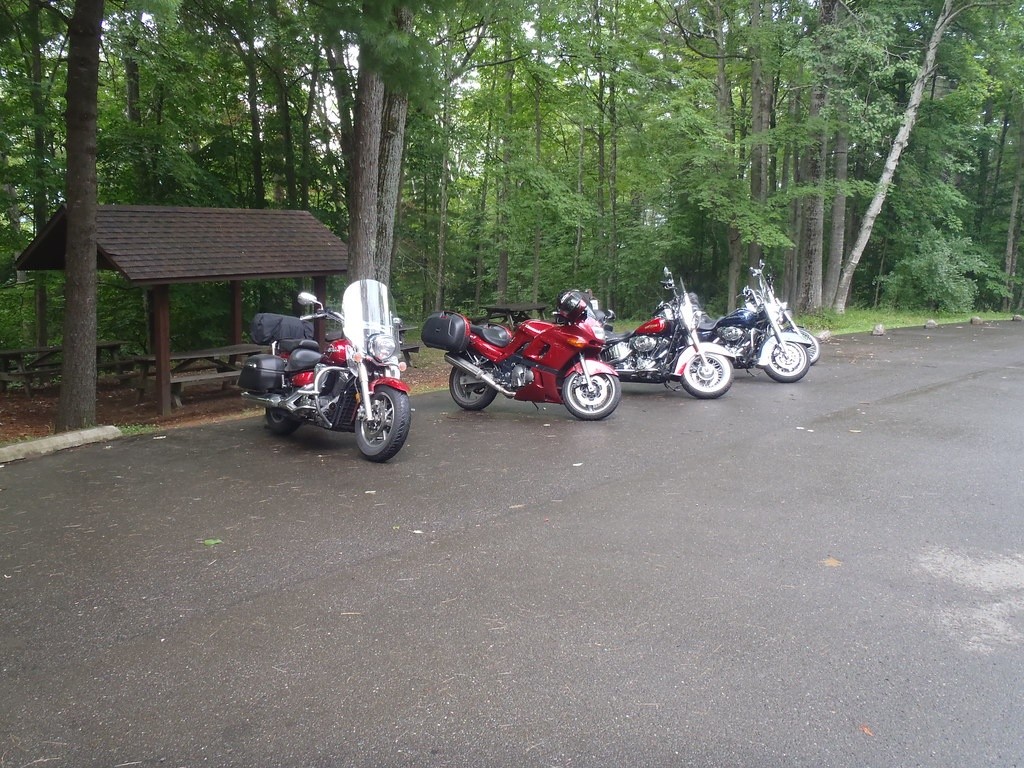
[558,290,589,322]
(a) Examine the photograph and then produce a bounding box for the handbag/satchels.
[251,313,314,346]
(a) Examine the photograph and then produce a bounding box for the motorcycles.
[421,288,621,420]
[551,259,820,399]
[238,279,411,463]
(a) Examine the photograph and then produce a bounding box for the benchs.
[400,344,420,353]
[170,369,242,394]
[468,313,518,324]
[10,360,136,383]
[8,362,62,370]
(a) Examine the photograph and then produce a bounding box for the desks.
[399,326,419,367]
[479,303,549,331]
[0,341,128,400]
[133,344,261,409]
